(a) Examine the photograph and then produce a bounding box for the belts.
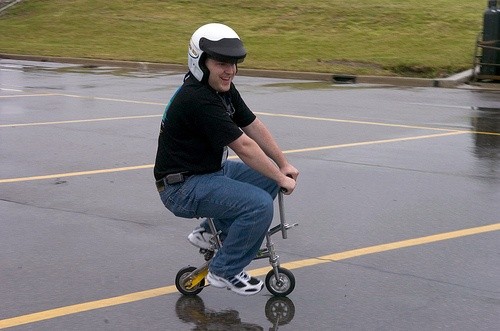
[155,172,189,188]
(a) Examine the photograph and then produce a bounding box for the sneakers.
[205,263,264,295]
[188,227,224,253]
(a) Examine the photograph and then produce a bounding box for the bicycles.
[174,173,300,297]
[175,294,296,331]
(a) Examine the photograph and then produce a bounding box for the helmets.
[188,22,247,82]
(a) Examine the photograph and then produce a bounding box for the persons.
[154,22,299,296]
